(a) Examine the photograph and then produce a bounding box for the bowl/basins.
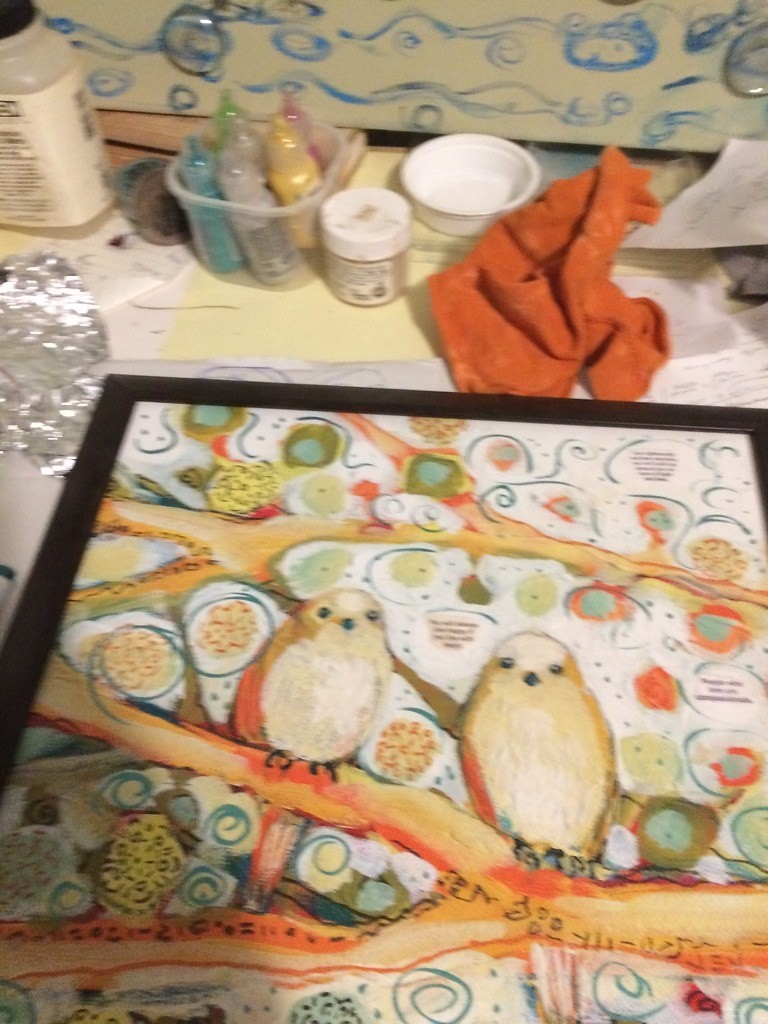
[398,132,543,235]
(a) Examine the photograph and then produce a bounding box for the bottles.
[319,185,411,306]
[0,0,118,238]
[176,85,337,287]
